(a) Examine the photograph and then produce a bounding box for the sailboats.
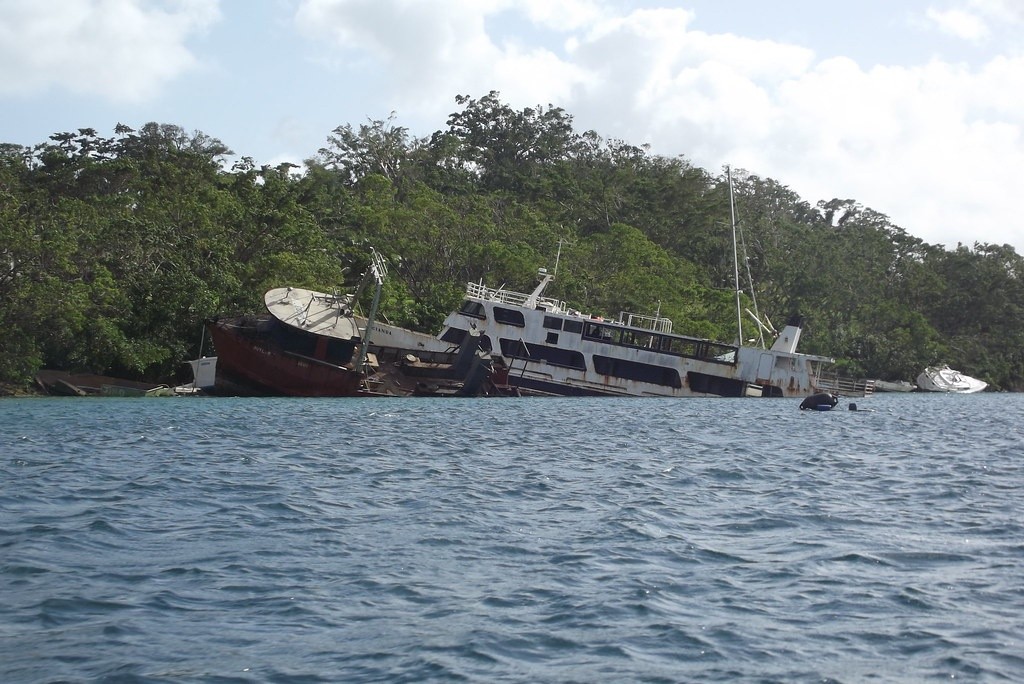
[434,164,839,400]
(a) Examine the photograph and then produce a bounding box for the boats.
[202,243,533,397]
[917,365,988,395]
